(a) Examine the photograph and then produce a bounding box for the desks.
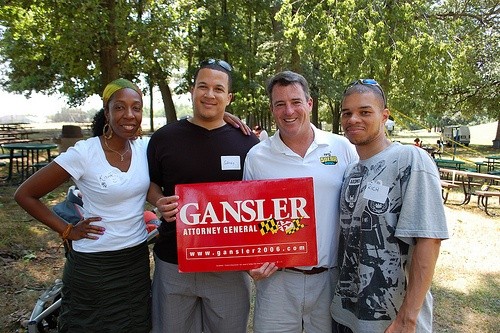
[486,156,500,171]
[422,147,433,152]
[439,168,500,209]
[435,160,465,181]
[1,143,58,185]
[0,123,31,130]
[0,130,39,139]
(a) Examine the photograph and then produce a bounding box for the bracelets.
[62,224,72,239]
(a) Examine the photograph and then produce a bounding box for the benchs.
[0,126,49,174]
[432,161,500,217]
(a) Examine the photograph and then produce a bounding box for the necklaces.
[104,140,130,162]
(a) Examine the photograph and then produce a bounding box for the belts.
[284,266,337,275]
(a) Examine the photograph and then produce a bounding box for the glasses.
[343,79,386,108]
[199,58,232,72]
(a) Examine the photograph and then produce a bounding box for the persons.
[147,58,260,333]
[414,138,420,147]
[255,126,268,141]
[330,79,449,333]
[433,140,443,158]
[242,71,360,333]
[14,78,251,333]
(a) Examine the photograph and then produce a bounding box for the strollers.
[28,186,161,333]
